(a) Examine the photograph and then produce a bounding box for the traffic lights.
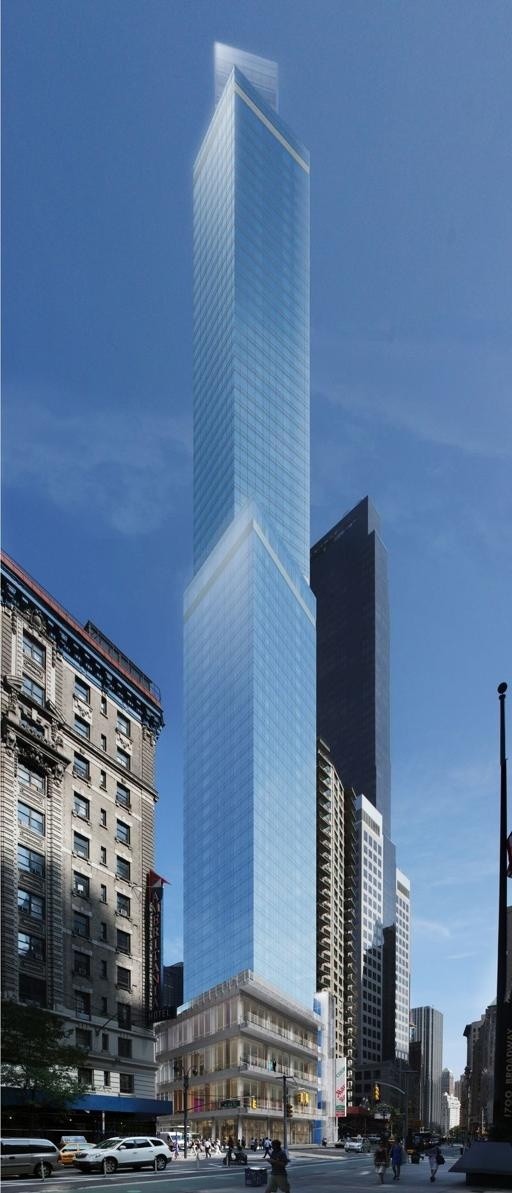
[288,1103,295,1117]
[252,1099,256,1109]
[297,1093,303,1103]
[376,1086,379,1100]
[306,1092,309,1106]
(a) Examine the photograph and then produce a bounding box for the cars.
[336,1134,381,1153]
[1,1135,173,1178]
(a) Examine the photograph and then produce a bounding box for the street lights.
[173,1063,198,1160]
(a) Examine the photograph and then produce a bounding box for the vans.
[160,1131,182,1151]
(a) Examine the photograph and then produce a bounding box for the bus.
[156,1125,193,1150]
[405,1130,441,1153]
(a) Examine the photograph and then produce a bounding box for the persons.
[263,1139,291,1193]
[171,1135,272,1161]
[374,1132,441,1184]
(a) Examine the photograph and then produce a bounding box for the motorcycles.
[222,1146,248,1166]
